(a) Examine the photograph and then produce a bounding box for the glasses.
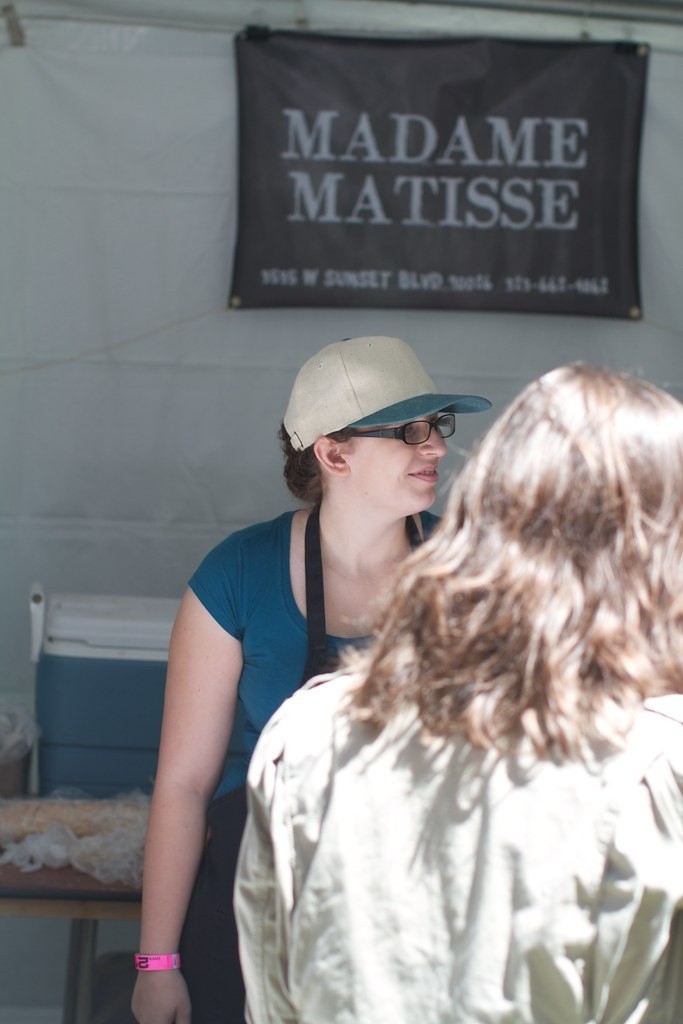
[342,405,457,445]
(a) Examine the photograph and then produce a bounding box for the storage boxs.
[34,591,181,801]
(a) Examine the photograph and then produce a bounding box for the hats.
[283,335,491,451]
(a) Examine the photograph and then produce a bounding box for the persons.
[230,362,683,1024]
[129,335,459,1024]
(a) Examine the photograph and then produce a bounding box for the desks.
[0,850,141,1024]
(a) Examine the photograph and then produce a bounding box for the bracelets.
[135,952,181,971]
